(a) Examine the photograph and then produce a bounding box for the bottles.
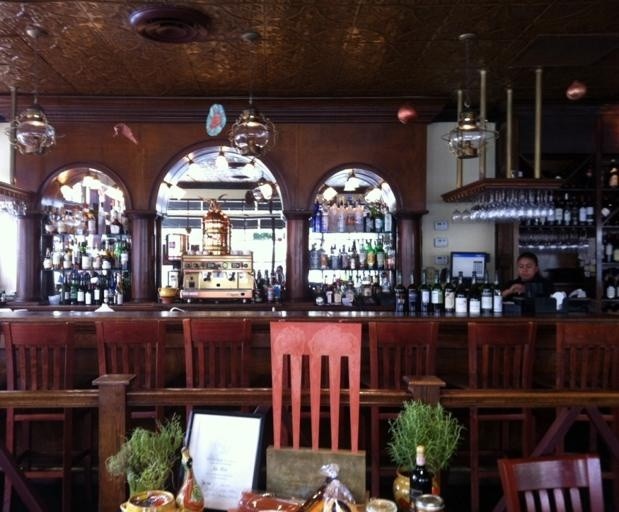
[555,193,593,226]
[394,269,504,315]
[600,201,614,219]
[177,446,205,512]
[609,159,618,187]
[1,289,6,305]
[409,444,433,511]
[599,233,619,299]
[308,195,396,270]
[43,199,129,305]
[189,276,194,290]
[252,267,284,303]
[322,270,390,305]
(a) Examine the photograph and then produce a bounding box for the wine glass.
[519,227,593,254]
[451,192,556,224]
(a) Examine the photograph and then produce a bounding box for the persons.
[497,252,553,298]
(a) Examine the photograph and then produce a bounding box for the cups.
[366,497,398,511]
[315,296,323,306]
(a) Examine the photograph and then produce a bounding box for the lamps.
[4,27,66,155]
[129,6,211,43]
[445,32,499,163]
[226,31,279,160]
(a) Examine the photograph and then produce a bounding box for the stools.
[443,320,535,512]
[369,319,441,503]
[2,318,97,512]
[496,453,606,510]
[174,317,249,492]
[97,319,168,450]
[543,319,617,489]
[267,320,367,502]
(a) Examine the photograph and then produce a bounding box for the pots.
[157,287,179,297]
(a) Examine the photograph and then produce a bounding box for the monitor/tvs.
[451,252,486,287]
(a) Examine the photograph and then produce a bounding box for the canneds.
[416,494,445,512]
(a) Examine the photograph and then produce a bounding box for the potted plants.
[106,412,184,509]
[387,390,467,510]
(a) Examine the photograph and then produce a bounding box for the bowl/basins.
[126,489,176,512]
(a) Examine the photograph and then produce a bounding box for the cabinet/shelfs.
[595,163,619,299]
[306,167,395,305]
[36,167,132,303]
